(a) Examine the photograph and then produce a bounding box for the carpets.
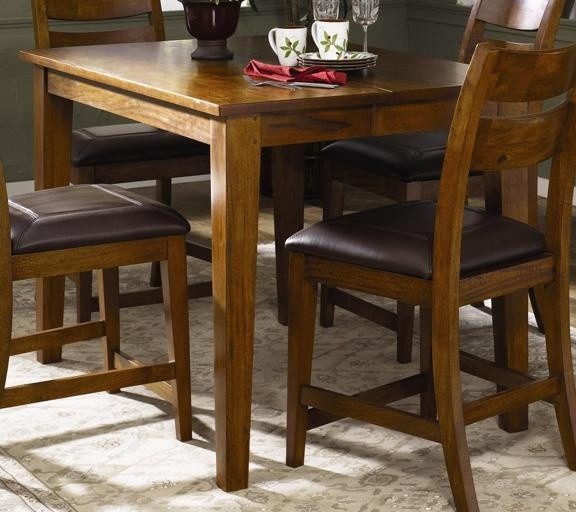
[0,243,576,512]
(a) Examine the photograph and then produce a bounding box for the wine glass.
[312,0,340,21]
[180,0,244,59]
[349,0,381,74]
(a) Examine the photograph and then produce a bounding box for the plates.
[299,49,380,71]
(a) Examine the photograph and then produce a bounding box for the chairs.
[284,41,576,512]
[0,157,192,442]
[320,0,568,363]
[31,0,212,323]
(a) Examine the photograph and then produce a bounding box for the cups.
[268,26,308,68]
[311,20,350,61]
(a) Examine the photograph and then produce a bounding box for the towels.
[244,60,347,85]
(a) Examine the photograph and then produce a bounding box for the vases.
[178,0,244,60]
